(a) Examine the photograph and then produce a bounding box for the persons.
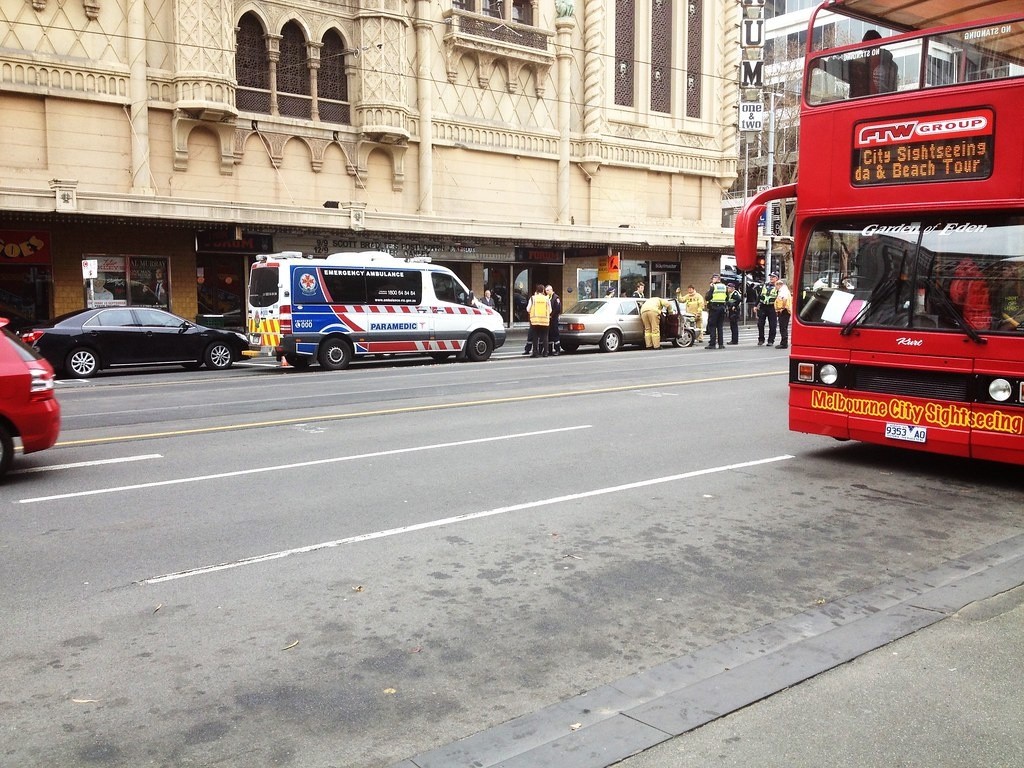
[641,297,673,349]
[440,276,465,303]
[727,283,742,345]
[754,272,779,346]
[490,288,502,311]
[87,273,113,301]
[774,281,792,349]
[735,283,763,321]
[605,287,616,299]
[143,268,167,304]
[633,282,644,298]
[277,351,289,366]
[807,30,898,98]
[480,290,495,310]
[676,285,704,342]
[705,273,730,349]
[619,288,627,297]
[513,289,529,322]
[583,277,593,299]
[522,284,561,357]
[923,233,1024,332]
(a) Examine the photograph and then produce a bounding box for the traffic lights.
[758,258,765,276]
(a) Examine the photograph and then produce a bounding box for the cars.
[557,297,711,353]
[96,279,169,311]
[813,278,854,293]
[493,286,527,310]
[10,306,250,379]
[0,318,60,478]
[706,272,764,304]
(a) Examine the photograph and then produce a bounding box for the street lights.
[763,88,792,273]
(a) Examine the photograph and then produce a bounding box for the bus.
[734,0,1024,463]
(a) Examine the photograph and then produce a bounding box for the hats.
[711,273,720,279]
[728,283,735,287]
[608,286,615,292]
[768,272,779,279]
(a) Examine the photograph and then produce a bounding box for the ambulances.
[245,251,506,370]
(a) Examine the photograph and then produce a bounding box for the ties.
[157,281,162,298]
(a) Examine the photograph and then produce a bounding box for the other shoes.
[549,352,553,355]
[530,355,538,357]
[543,354,548,357]
[554,351,561,355]
[704,346,715,349]
[766,342,772,346]
[757,341,763,345]
[539,353,542,356]
[776,344,788,349]
[522,351,530,354]
[647,346,663,350]
[727,341,738,344]
[719,345,725,349]
